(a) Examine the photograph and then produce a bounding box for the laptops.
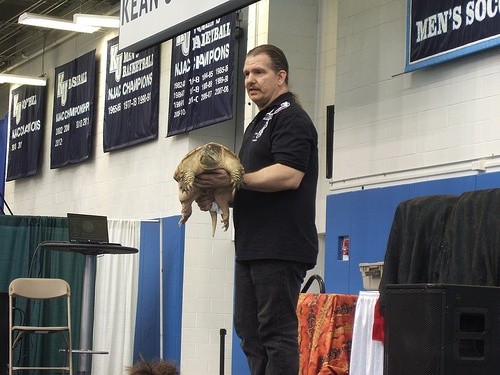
[67,213,121,246]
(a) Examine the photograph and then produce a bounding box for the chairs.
[7,277,73,375]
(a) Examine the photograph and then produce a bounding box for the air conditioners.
[15,10,101,33]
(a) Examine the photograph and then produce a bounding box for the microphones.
[248,102,251,105]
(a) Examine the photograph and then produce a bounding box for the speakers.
[384,282,499,375]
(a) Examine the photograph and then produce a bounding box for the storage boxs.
[359,261,384,290]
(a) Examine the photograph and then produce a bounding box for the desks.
[349,290,384,375]
[299,293,359,375]
[39,242,140,375]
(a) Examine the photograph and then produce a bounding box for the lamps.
[73,0,121,29]
[0,28,49,87]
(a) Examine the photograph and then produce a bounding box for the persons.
[191,43,319,375]
